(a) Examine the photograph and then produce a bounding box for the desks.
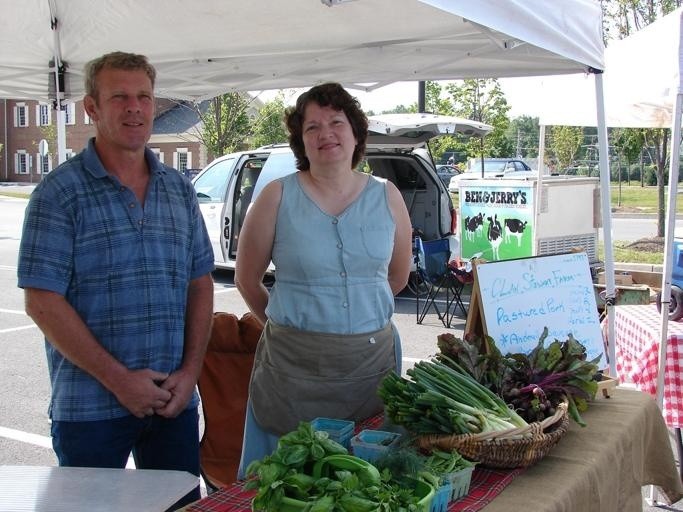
[174,389,683,512]
[0,465,200,512]
[599,304,683,482]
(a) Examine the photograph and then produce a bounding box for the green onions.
[375,351,529,440]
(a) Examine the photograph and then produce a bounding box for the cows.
[504,218,528,247]
[464,212,485,243]
[487,213,503,261]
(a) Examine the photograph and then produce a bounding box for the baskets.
[412,392,569,469]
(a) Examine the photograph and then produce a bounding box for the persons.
[446,155,453,165]
[234,81,414,480]
[16,51,217,512]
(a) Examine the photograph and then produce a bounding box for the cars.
[447,158,539,195]
[433,164,463,189]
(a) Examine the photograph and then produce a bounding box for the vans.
[155,113,497,267]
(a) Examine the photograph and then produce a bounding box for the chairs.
[194,311,263,492]
[240,186,254,227]
[414,235,474,328]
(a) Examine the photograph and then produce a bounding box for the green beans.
[427,447,478,475]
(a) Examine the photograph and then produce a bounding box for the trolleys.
[655,240,683,321]
